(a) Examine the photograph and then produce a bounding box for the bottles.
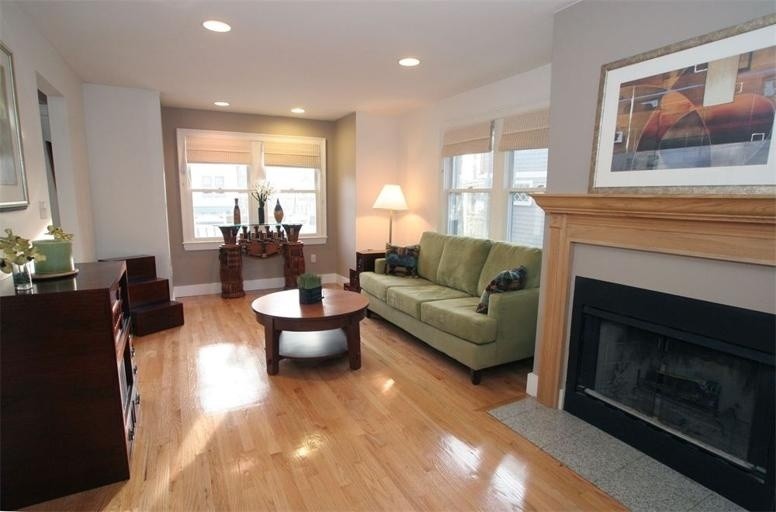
[274,199,283,223]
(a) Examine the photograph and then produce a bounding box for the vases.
[258,207,265,225]
[273,197,284,223]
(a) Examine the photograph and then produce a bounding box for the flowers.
[251,181,277,208]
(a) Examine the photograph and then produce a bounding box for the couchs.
[360,231,543,385]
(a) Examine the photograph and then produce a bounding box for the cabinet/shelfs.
[352,248,385,292]
[0,260,140,511]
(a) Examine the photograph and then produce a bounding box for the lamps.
[372,185,410,244]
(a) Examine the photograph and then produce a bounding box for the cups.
[32,240,74,273]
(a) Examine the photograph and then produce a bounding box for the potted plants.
[31,225,77,273]
[295,272,325,305]
[0,228,48,290]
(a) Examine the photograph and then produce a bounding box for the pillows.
[474,263,527,314]
[384,242,421,280]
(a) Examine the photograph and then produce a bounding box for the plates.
[31,270,78,279]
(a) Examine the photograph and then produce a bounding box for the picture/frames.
[585,11,776,196]
[0,40,31,212]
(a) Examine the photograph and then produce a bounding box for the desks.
[218,223,306,299]
